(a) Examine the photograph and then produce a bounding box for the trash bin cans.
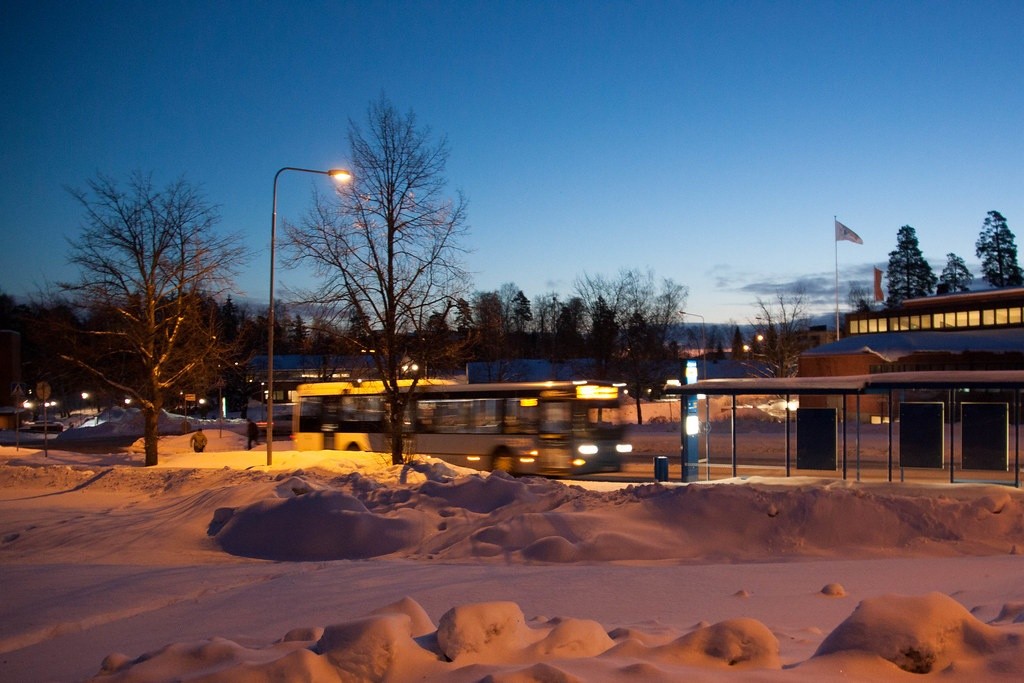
[654,456,669,482]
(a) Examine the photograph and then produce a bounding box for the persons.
[190,428,208,453]
[247,418,260,450]
[33,406,40,421]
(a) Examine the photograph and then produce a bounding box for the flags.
[835,221,863,244]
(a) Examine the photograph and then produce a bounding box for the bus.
[290,378,624,478]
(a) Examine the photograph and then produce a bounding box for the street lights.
[679,310,712,481]
[267,166,351,465]
